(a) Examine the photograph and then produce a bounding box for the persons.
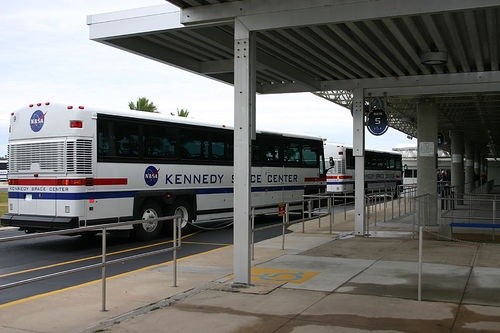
[436,169,450,181]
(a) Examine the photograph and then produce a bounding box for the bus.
[7,103,336,238]
[319,140,406,199]
